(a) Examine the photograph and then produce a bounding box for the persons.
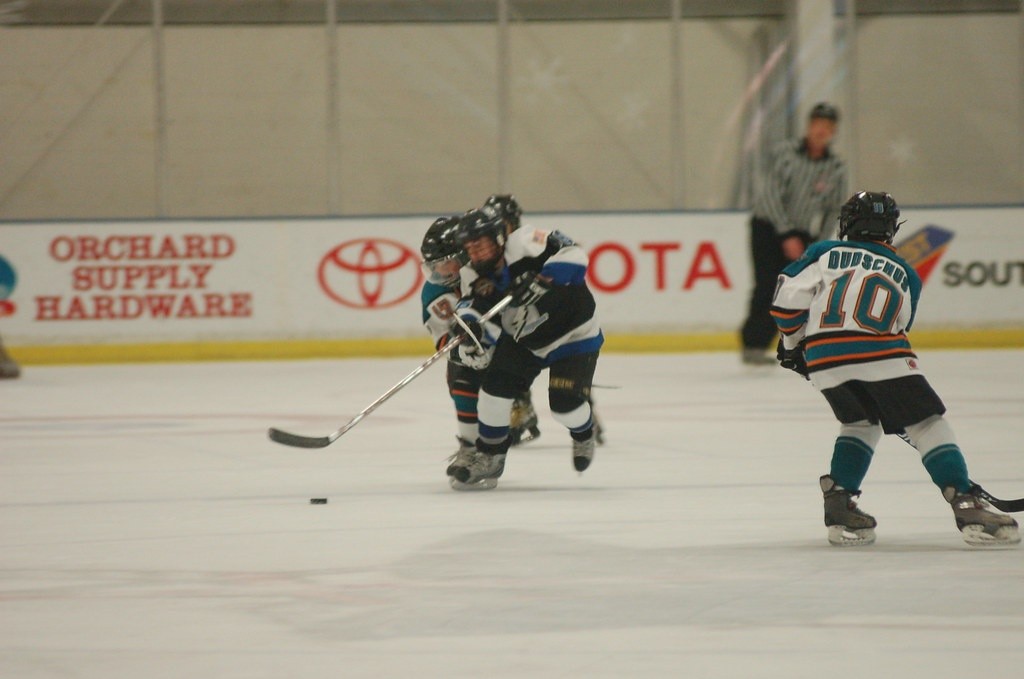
[421,192,1024,546]
[742,103,847,364]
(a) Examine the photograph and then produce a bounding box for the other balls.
[309,497,329,505]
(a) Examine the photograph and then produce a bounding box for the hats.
[811,105,837,120]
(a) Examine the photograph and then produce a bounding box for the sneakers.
[820,475,877,547]
[942,485,1021,550]
[509,389,540,447]
[570,423,594,471]
[451,432,512,490]
[443,434,480,483]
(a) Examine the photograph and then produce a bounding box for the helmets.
[456,209,505,275]
[484,194,522,232]
[839,192,907,245]
[421,217,466,265]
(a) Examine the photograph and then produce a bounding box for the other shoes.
[743,348,775,364]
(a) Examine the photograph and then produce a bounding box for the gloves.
[452,318,485,346]
[509,271,552,307]
[777,335,810,380]
[448,337,496,370]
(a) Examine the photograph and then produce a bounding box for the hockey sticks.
[268,291,515,449]
[893,431,1024,513]
[451,311,487,357]
